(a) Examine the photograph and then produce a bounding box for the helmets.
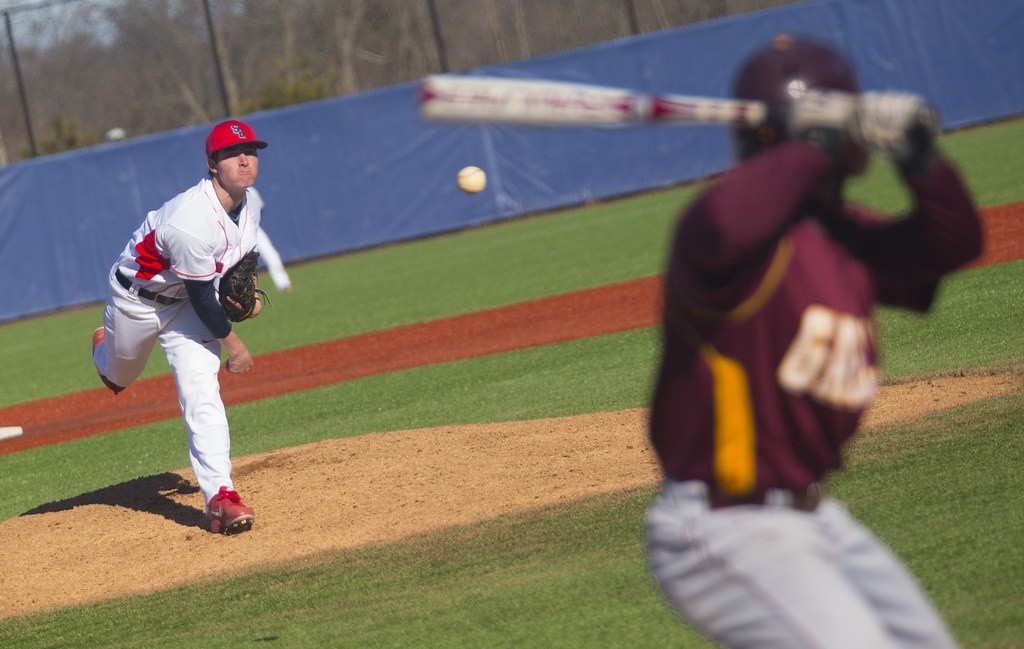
[734,37,870,174]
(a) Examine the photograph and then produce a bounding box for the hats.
[206,120,268,157]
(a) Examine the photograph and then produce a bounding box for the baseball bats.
[421,71,939,137]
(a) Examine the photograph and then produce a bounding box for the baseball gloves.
[217,253,275,323]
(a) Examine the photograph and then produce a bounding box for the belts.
[115,269,182,305]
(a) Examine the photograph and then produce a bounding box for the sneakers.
[207,486,255,535]
[92,327,126,395]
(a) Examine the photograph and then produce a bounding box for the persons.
[256,227,292,290]
[646,35,982,649]
[92,120,267,536]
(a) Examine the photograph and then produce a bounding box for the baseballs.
[458,165,486,197]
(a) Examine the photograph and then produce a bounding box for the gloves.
[859,90,942,157]
[788,90,855,133]
